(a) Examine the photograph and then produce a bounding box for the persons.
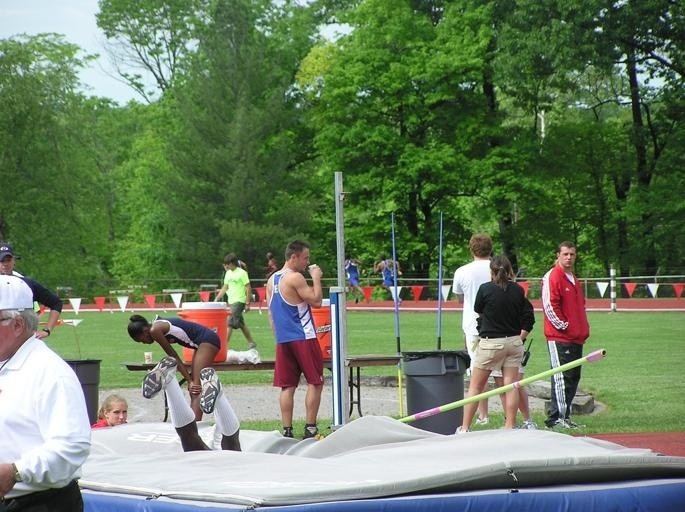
[266,240,323,440]
[213,254,257,348]
[43,328,50,336]
[266,253,277,271]
[142,357,240,451]
[455,256,536,435]
[90,395,127,427]
[543,241,590,432]
[1,275,90,512]
[1,242,62,339]
[345,254,403,307]
[453,235,537,430]
[127,314,221,421]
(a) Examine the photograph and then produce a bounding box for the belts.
[481,336,512,338]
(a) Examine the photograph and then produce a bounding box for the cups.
[308,264,318,271]
[144,352,152,364]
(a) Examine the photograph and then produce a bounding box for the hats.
[0,275,34,310]
[0,244,13,261]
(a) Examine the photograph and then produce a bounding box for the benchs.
[127,360,331,423]
[345,355,400,417]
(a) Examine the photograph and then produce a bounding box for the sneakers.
[199,368,219,414]
[455,418,578,435]
[143,357,177,399]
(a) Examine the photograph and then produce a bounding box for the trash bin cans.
[64,360,102,427]
[402,351,471,435]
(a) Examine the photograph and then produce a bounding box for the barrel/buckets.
[177,301,233,363]
[310,299,332,360]
